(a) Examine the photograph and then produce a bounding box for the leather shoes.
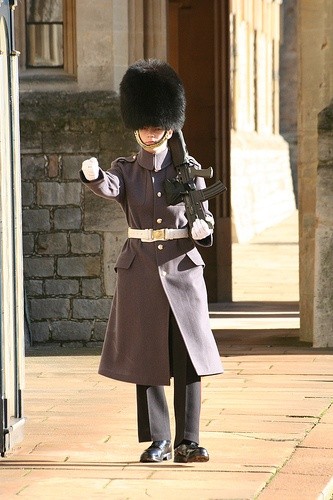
[139,439,173,463]
[174,442,209,463]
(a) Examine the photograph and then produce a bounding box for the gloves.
[190,218,214,241]
[81,156,100,182]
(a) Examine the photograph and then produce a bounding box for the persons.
[78,59,223,464]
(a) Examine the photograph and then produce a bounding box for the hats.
[119,58,187,132]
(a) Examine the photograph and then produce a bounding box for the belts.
[127,227,189,243]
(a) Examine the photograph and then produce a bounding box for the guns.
[169,124,227,233]
